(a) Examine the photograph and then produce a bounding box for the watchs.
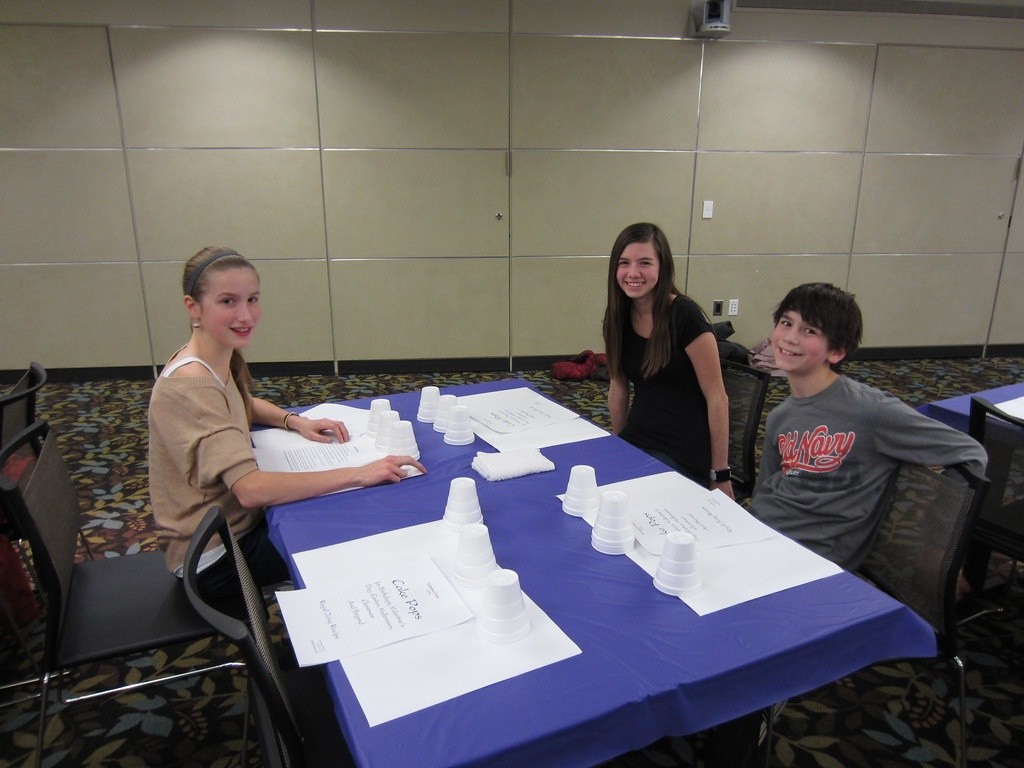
[709,466,731,482]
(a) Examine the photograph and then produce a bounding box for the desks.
[250,379,936,767]
[914,380,1024,620]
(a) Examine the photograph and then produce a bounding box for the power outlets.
[713,300,723,316]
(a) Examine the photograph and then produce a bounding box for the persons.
[640,281,987,768]
[146,245,428,611]
[602,222,736,504]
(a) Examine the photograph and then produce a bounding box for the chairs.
[954,395,1024,627]
[757,461,967,767]
[182,505,355,768]
[0,362,256,768]
[722,361,770,503]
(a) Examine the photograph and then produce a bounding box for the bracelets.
[284,412,299,430]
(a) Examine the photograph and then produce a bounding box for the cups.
[482,569,529,642]
[591,491,635,555]
[454,523,496,587]
[433,394,457,433]
[389,420,421,461]
[562,465,599,517]
[417,386,440,423]
[444,406,475,445]
[375,411,400,450]
[443,477,483,531]
[653,531,701,596]
[367,399,390,437]
